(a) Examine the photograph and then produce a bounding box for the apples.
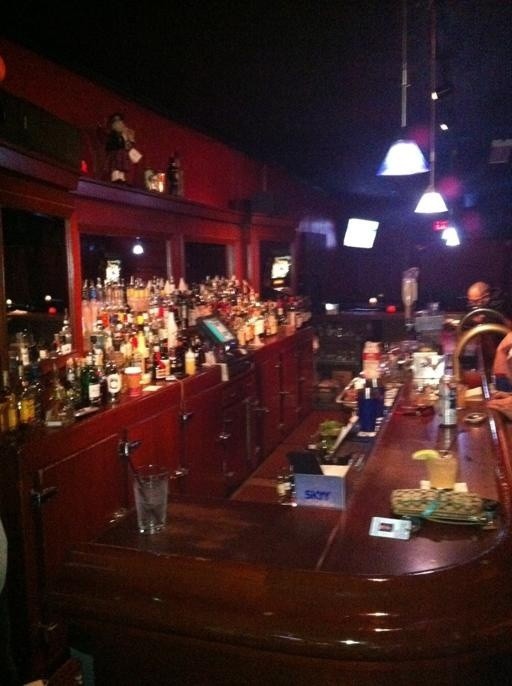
[48,307,57,314]
[387,305,396,313]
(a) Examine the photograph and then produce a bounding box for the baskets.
[388,486,499,527]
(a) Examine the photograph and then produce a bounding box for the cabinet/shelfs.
[0,330,314,686]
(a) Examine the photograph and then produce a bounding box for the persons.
[102,109,132,189]
[458,281,506,373]
[485,391,511,424]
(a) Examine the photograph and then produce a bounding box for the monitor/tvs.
[342,218,380,250]
[196,315,237,343]
[268,254,292,280]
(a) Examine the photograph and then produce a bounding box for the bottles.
[0,274,302,435]
[439,352,457,427]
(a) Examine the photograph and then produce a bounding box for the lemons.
[412,449,439,461]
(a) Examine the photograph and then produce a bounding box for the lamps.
[375,0,462,248]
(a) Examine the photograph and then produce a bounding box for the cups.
[425,450,460,491]
[132,464,169,537]
[357,378,385,432]
